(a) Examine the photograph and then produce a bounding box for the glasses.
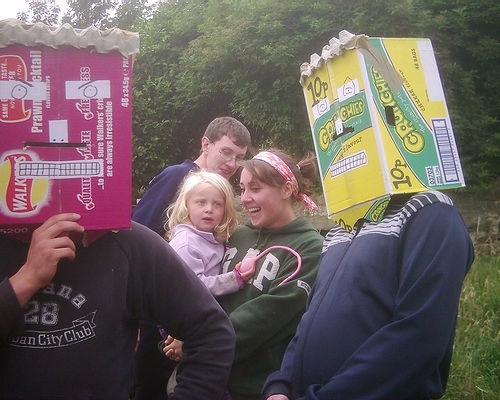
[213,142,244,164]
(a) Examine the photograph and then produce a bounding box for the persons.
[158,148,325,400]
[129,117,254,368]
[261,30,476,400]
[0,18,237,400]
